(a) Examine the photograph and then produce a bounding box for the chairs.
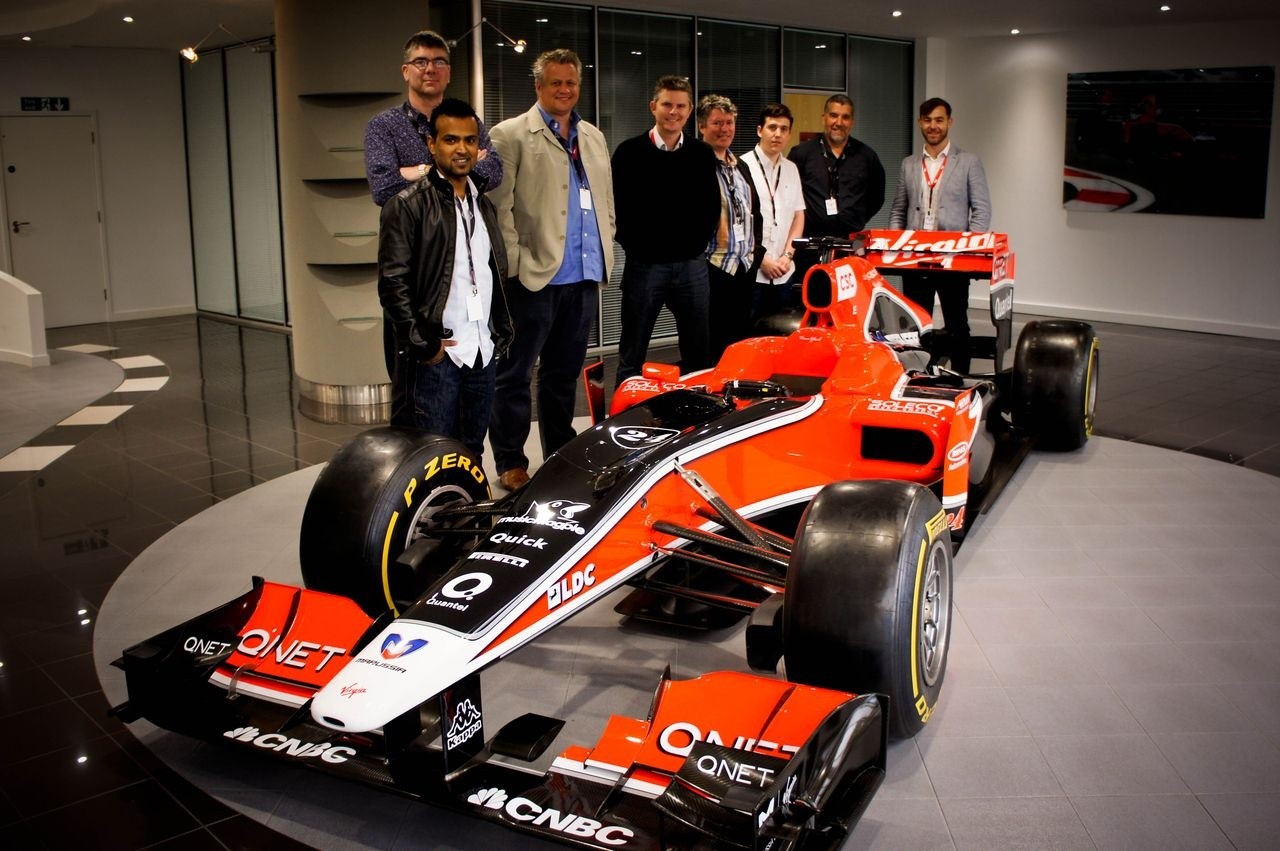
[764,328,837,398]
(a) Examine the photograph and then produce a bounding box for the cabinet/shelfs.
[275,0,427,425]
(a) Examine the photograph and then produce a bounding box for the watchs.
[418,164,426,176]
[784,250,794,259]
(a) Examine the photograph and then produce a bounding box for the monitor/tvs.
[1062,65,1275,219]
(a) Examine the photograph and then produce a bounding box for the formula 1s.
[89,225,1106,851]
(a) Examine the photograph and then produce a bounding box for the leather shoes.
[500,469,530,492]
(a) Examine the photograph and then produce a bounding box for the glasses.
[403,58,449,71]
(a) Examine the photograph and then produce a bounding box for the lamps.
[450,17,527,53]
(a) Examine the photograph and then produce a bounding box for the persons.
[886,97,992,376]
[737,103,807,336]
[488,47,616,492]
[377,97,515,463]
[611,75,721,392]
[788,94,885,313]
[695,94,768,358]
[363,30,503,381]
